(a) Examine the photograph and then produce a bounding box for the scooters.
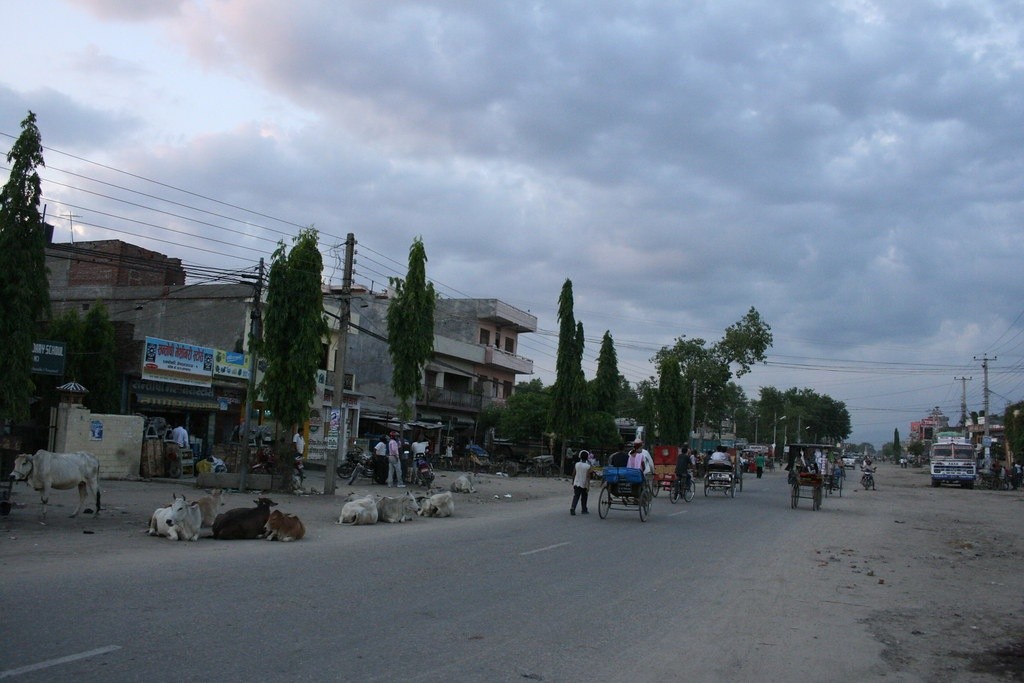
[859,465,877,490]
[247,446,304,491]
[864,459,872,466]
[335,444,434,489]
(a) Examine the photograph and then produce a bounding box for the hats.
[635,439,643,443]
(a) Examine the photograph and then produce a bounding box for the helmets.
[380,434,390,442]
[390,431,400,438]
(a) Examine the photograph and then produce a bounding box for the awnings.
[136,392,220,411]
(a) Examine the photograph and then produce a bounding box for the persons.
[674,447,693,500]
[1011,463,1022,490]
[388,431,406,487]
[411,435,431,484]
[565,444,577,475]
[710,445,732,461]
[704,451,712,463]
[688,449,697,464]
[446,440,453,468]
[570,450,592,515]
[293,427,306,457]
[608,443,629,467]
[755,454,764,479]
[173,422,190,449]
[800,462,821,486]
[627,438,650,504]
[900,458,908,468]
[861,456,875,490]
[993,459,1009,476]
[375,436,387,457]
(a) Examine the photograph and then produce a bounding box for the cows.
[257,510,305,542]
[335,495,379,526]
[200,489,224,526]
[416,491,454,518]
[202,496,278,540]
[8,449,101,519]
[150,492,202,542]
[450,476,477,494]
[376,490,421,523]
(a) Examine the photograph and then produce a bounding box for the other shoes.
[388,484,393,488]
[570,508,575,515]
[582,511,589,514]
[397,484,406,487]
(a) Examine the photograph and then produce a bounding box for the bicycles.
[525,456,561,479]
[668,473,696,504]
[769,460,776,473]
[977,473,1021,491]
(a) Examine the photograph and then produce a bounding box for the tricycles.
[784,439,846,512]
[596,466,654,522]
[704,460,743,499]
[652,446,691,497]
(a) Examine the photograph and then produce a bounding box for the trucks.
[842,453,855,471]
[929,437,975,489]
[738,445,765,467]
